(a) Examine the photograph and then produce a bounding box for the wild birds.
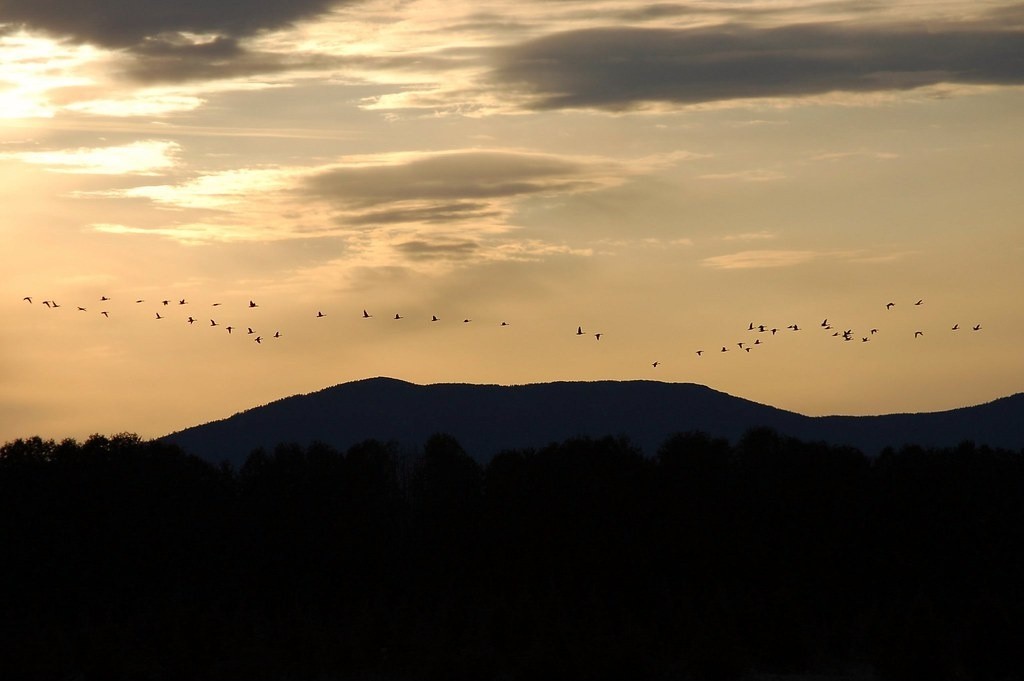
[248,328,256,334]
[179,299,188,304]
[162,301,171,305]
[886,303,895,310]
[23,296,32,303]
[249,300,259,307]
[187,317,197,324]
[101,311,109,318]
[595,334,603,340]
[787,324,802,330]
[973,324,982,330]
[822,319,879,343]
[77,307,86,312]
[136,300,144,303]
[696,351,704,356]
[915,299,923,305]
[736,323,780,352]
[393,314,403,319]
[914,331,924,338]
[652,361,660,367]
[317,312,326,317]
[211,319,219,326]
[42,301,60,308]
[100,296,110,301]
[212,303,221,306]
[226,327,235,333]
[362,309,372,318]
[431,316,440,321]
[464,319,472,322]
[501,322,509,326]
[951,324,960,330]
[721,347,729,352]
[576,326,586,335]
[155,312,164,319]
[255,337,263,343]
[273,332,282,337]
